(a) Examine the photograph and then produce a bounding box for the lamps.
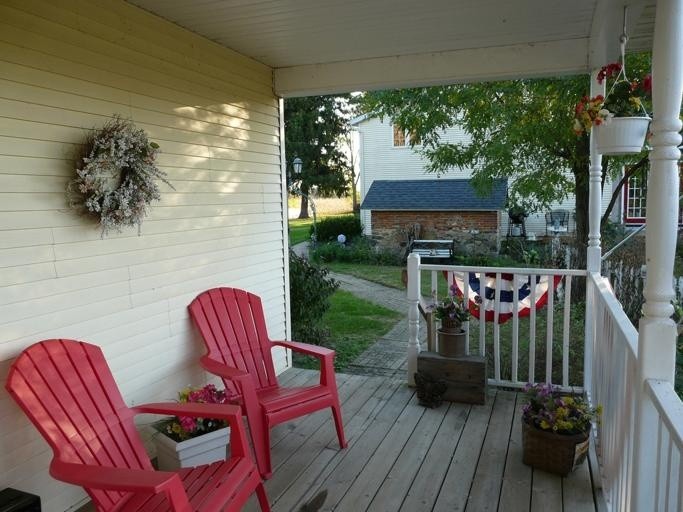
[286,154,304,189]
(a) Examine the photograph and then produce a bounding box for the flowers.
[146,375,233,446]
[517,372,603,433]
[67,105,177,241]
[567,56,656,141]
[419,278,481,332]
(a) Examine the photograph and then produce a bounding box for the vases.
[436,327,466,358]
[591,116,650,153]
[518,416,591,477]
[147,420,230,473]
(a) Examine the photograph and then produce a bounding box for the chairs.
[179,281,350,482]
[4,339,269,512]
[544,207,570,237]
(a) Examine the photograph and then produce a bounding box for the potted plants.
[506,206,528,237]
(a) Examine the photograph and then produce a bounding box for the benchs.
[402,238,456,267]
